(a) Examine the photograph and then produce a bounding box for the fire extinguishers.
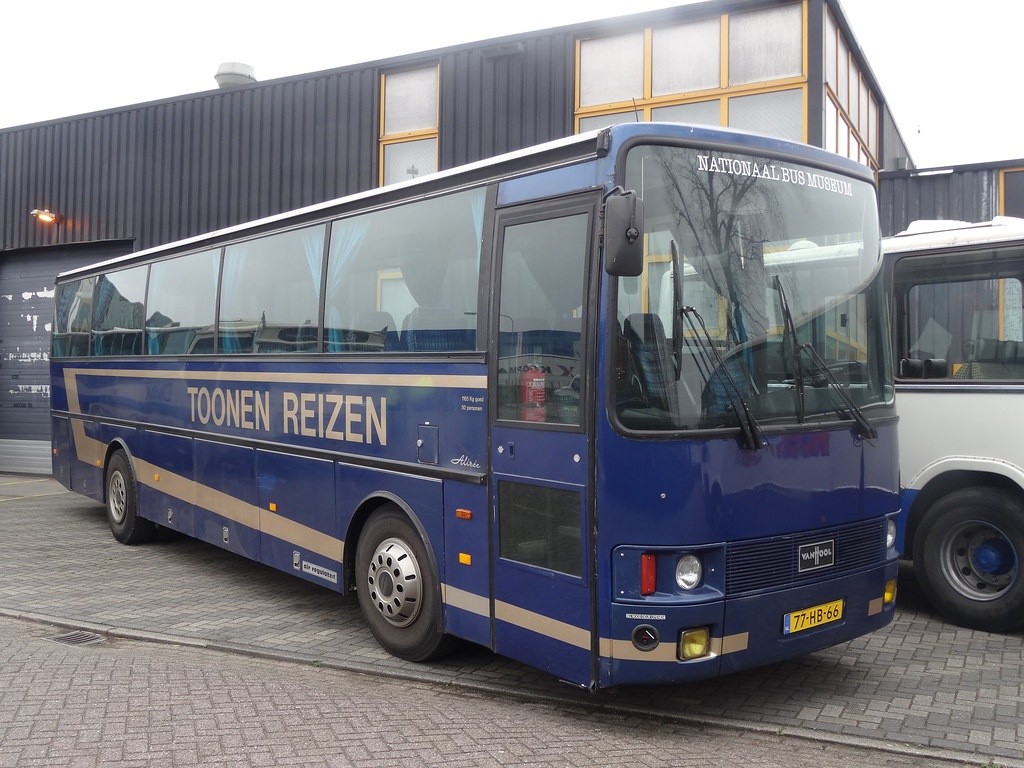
[519,363,546,424]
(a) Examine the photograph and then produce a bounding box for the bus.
[658,215,1024,634]
[48,119,905,696]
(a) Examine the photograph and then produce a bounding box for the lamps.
[31,208,56,222]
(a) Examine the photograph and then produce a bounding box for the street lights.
[464,310,515,400]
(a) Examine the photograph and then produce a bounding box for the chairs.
[73,308,410,352]
[899,358,948,379]
